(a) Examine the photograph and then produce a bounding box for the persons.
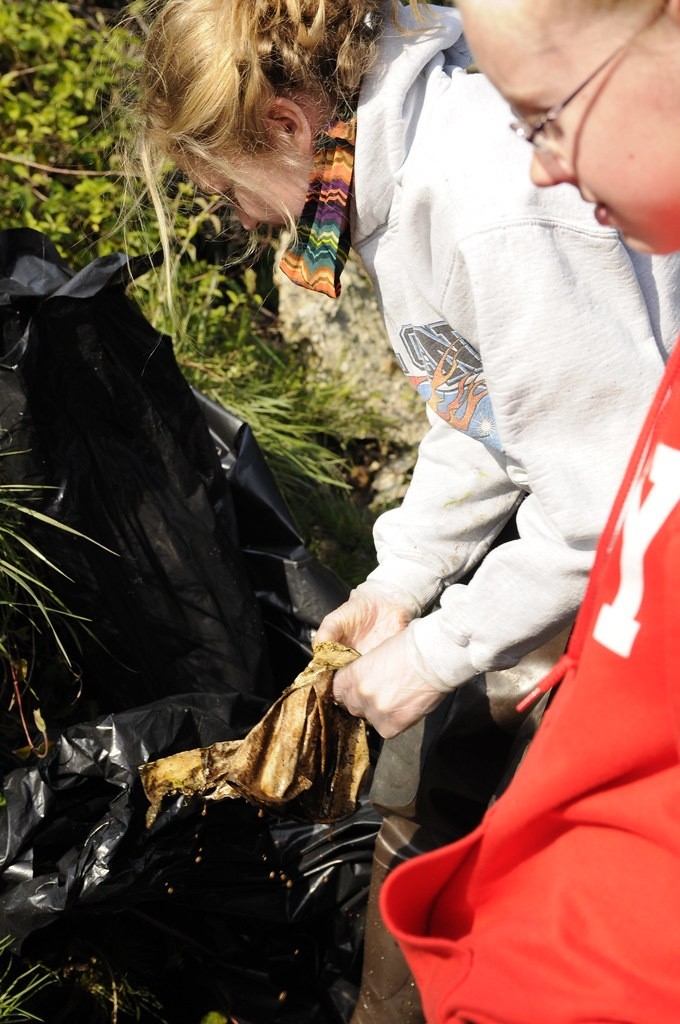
[379,1,680,1024]
[114,0,680,834]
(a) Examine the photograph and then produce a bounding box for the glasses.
[509,0,671,145]
